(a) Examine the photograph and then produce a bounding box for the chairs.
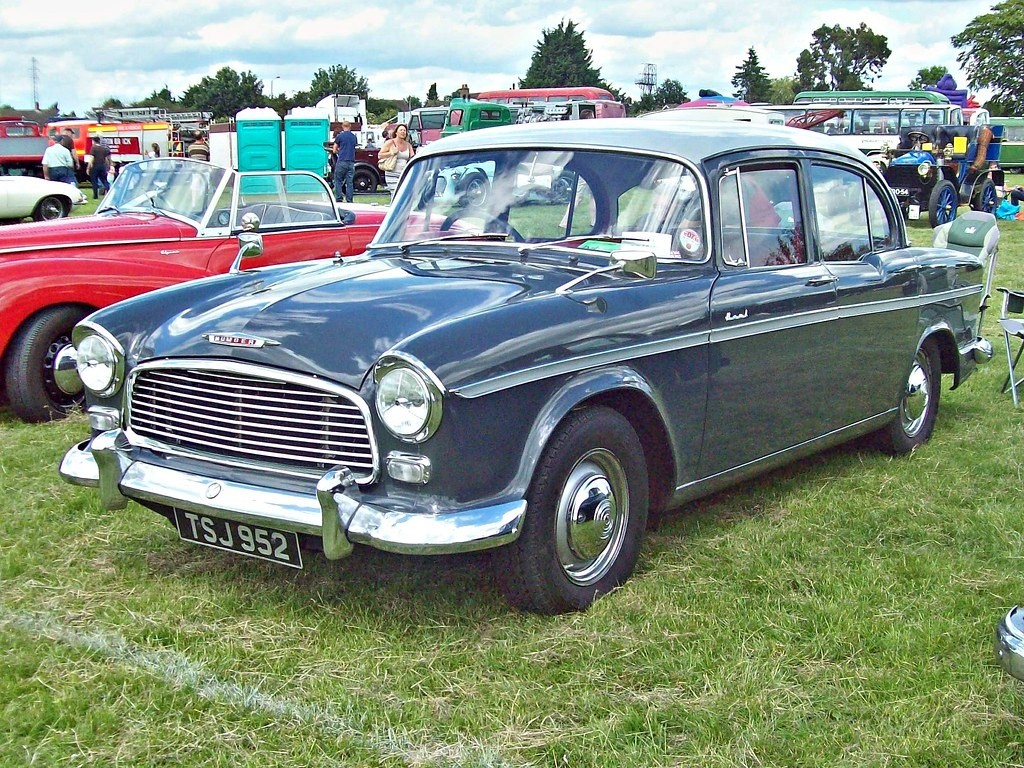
[262,206,331,226]
[995,286,1024,408]
[211,204,268,229]
[932,210,1001,336]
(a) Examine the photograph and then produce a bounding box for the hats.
[89,137,100,144]
[53,135,64,143]
[65,128,75,134]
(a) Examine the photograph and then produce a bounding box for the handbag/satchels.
[377,139,399,171]
[995,198,1022,221]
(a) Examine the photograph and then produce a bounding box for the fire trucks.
[42,122,187,184]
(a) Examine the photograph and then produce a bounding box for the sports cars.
[0,157,480,423]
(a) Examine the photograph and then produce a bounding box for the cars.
[57,121,990,614]
[0,176,81,222]
[866,126,1002,226]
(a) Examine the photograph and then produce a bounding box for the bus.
[792,91,951,105]
[728,104,964,158]
[478,88,616,101]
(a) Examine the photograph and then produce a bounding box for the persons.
[42,135,74,185]
[188,131,210,209]
[146,144,161,170]
[86,137,110,200]
[333,121,358,204]
[97,131,115,195]
[60,128,80,188]
[378,124,415,201]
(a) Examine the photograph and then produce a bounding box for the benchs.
[899,124,981,155]
